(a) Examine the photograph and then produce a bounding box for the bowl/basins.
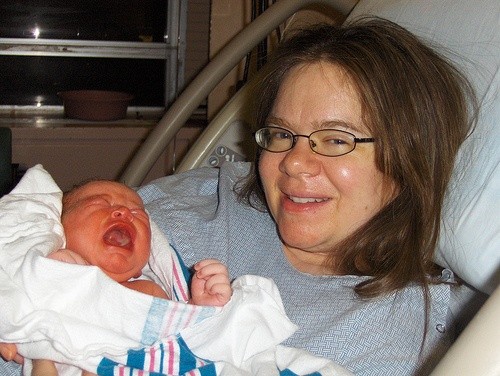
[57,90,133,121]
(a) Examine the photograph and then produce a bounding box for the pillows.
[342,0,500,296]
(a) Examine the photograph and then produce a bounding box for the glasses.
[250,125,378,158]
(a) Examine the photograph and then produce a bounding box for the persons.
[0,12,479,376]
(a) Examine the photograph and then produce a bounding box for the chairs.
[120,1,500,375]
